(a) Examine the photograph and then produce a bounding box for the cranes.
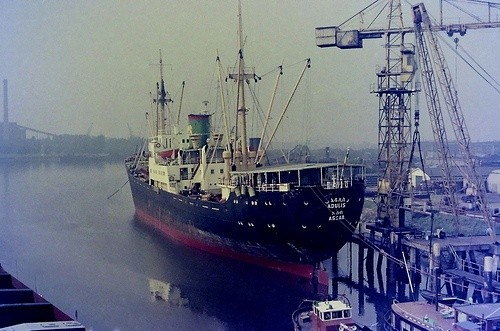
[314,0,500,233]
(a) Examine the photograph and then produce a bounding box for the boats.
[0,263,87,331]
[292,292,374,331]
[420,289,459,305]
[383,268,500,331]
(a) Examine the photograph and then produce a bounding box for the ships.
[124,0,367,286]
